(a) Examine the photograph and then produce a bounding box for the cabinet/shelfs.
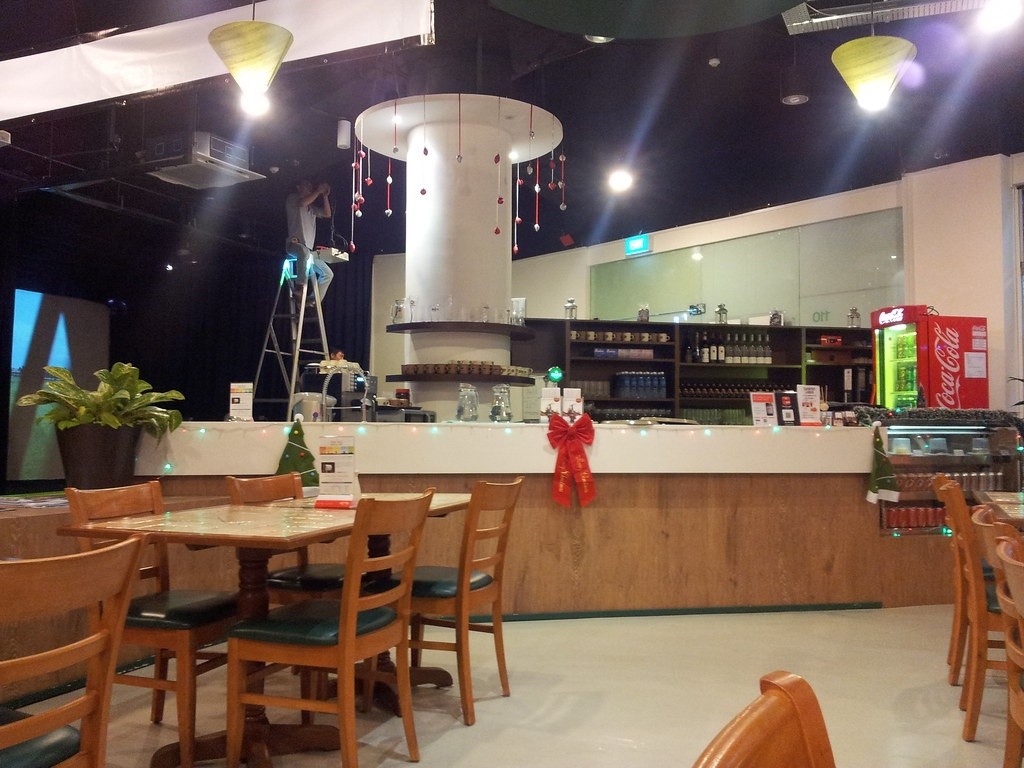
[805,325,874,415]
[565,317,678,421]
[384,322,537,388]
[676,324,805,421]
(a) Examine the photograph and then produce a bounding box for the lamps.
[830,2,918,115]
[779,32,811,105]
[209,0,296,117]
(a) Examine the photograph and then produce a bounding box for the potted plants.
[17,361,186,490]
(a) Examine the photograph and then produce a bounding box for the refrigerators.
[871,305,989,410]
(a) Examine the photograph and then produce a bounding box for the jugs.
[390,299,414,324]
[488,384,513,421]
[456,383,479,421]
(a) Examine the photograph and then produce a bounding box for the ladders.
[253,254,329,421]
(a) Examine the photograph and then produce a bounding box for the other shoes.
[293,282,303,300]
[308,296,316,308]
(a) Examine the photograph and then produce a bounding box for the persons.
[285,177,335,314]
[330,348,347,361]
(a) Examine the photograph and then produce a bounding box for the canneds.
[886,472,1005,528]
[395,388,410,405]
[765,401,775,416]
[895,331,917,409]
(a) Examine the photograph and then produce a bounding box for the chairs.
[0,528,154,768]
[363,473,527,726]
[227,469,371,698]
[65,480,252,768]
[226,484,437,768]
[691,668,836,768]
[930,472,1024,768]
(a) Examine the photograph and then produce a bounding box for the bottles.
[763,334,772,363]
[570,371,666,397]
[847,307,861,328]
[681,384,792,399]
[684,332,693,362]
[593,408,752,425]
[756,334,763,364]
[748,334,756,363]
[564,298,577,319]
[715,304,728,324]
[718,333,725,363]
[636,303,649,321]
[734,334,740,363]
[741,334,747,364]
[693,332,700,363]
[770,305,784,326]
[401,364,533,377]
[396,389,410,402]
[710,333,717,363]
[701,332,710,363]
[726,334,732,363]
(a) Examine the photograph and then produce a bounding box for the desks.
[52,503,357,768]
[971,490,1024,532]
[262,466,471,719]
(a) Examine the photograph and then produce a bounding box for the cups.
[658,334,670,343]
[571,331,579,339]
[605,332,615,341]
[622,333,634,341]
[641,332,652,341]
[586,331,597,340]
[429,304,525,326]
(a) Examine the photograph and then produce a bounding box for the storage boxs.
[819,334,842,345]
